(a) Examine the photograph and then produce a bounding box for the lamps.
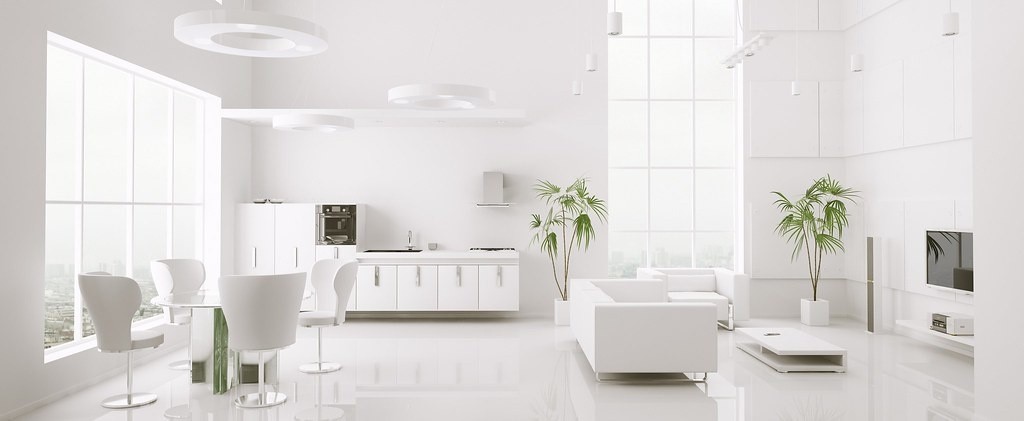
[942,0,958,36]
[606,0,622,36]
[851,0,864,72]
[585,0,599,72]
[572,79,583,96]
[791,0,802,96]
[720,0,773,69]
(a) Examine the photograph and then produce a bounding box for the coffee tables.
[735,327,848,373]
[895,320,974,360]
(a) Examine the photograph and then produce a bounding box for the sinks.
[362,250,423,253]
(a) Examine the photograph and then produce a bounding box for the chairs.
[297,258,358,374]
[77,271,165,410]
[150,259,206,371]
[217,272,308,409]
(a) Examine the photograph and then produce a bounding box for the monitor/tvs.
[926,231,974,295]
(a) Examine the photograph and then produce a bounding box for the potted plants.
[768,172,863,328]
[527,172,609,325]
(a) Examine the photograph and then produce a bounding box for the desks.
[151,288,314,395]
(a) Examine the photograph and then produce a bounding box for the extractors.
[474,171,509,208]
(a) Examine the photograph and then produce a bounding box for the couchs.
[570,278,718,383]
[637,267,749,331]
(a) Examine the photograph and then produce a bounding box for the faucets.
[408,230,412,250]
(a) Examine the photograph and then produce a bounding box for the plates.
[254,200,266,204]
[270,201,283,204]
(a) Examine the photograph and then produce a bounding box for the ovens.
[314,204,357,245]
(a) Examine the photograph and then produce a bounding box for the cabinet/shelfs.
[437,263,478,311]
[478,262,520,312]
[234,203,275,275]
[356,262,397,312]
[397,337,433,389]
[434,338,478,389]
[397,262,437,312]
[278,381,316,421]
[478,337,521,390]
[355,337,396,389]
[317,371,355,405]
[316,245,356,312]
[275,203,316,312]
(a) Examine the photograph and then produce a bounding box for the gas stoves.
[469,248,515,254]
